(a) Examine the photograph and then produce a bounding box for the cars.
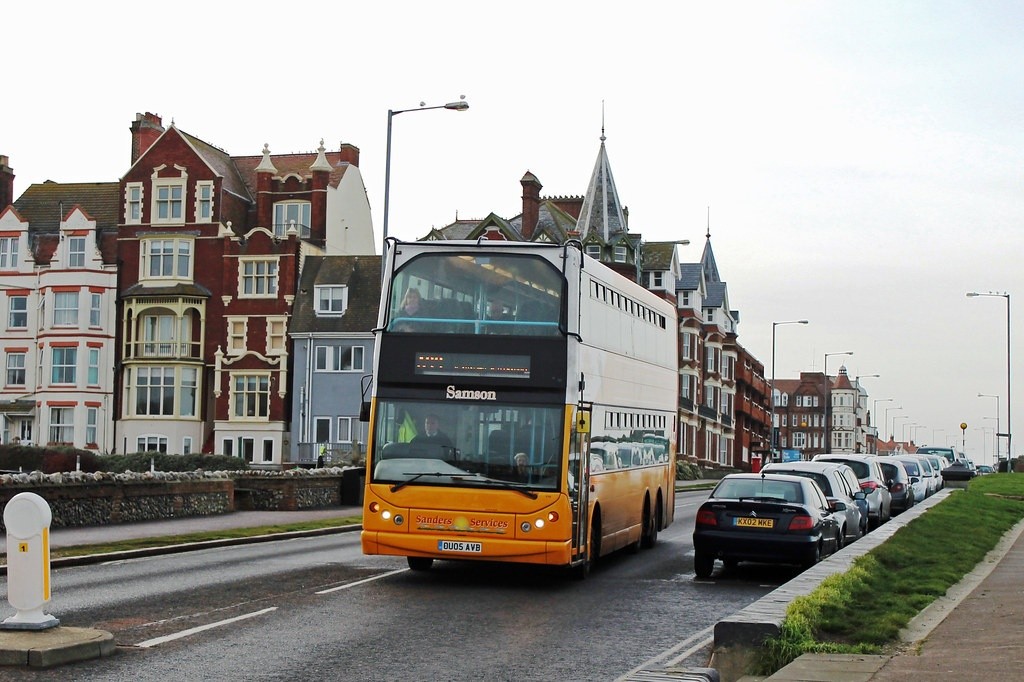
[893,445,998,505]
[757,461,875,544]
[692,473,847,579]
[810,453,895,532]
[876,457,920,514]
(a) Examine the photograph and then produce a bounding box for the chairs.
[393,297,560,334]
[737,485,755,498]
[482,426,665,471]
[783,490,796,500]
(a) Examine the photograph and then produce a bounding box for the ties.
[430,433,433,437]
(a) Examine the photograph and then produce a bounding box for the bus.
[360,237,680,581]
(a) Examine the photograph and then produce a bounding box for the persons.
[488,300,513,335]
[392,287,440,332]
[410,413,456,461]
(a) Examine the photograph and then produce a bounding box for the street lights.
[885,407,944,445]
[975,394,1000,468]
[769,320,808,444]
[824,351,853,453]
[966,292,1011,473]
[380,93,470,281]
[855,375,880,452]
[873,399,893,453]
